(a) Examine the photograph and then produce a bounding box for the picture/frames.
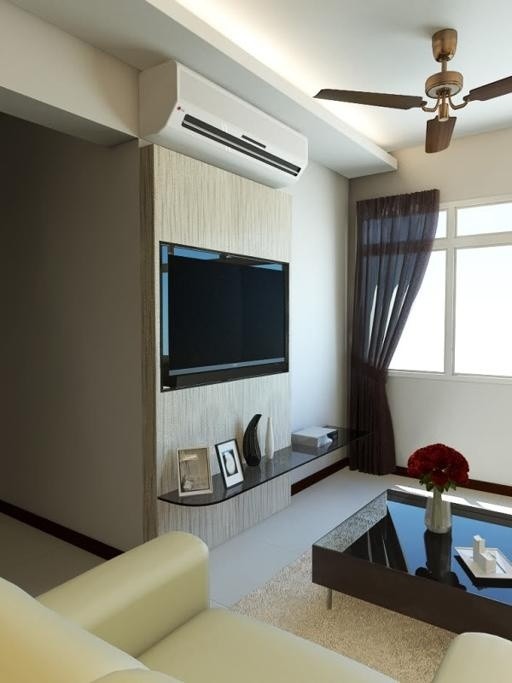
[176,446,212,497]
[214,439,246,488]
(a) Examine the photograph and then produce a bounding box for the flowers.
[406,440,471,496]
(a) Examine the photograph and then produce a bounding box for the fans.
[313,27,511,154]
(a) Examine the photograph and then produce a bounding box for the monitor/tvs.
[159,241,289,393]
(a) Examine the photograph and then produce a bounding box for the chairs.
[1,531,512,683]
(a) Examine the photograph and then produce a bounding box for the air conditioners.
[138,59,308,189]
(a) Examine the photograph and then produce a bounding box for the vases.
[422,492,453,534]
[241,411,262,466]
[423,529,455,573]
[264,416,274,459]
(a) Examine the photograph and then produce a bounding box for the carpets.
[231,485,512,682]
